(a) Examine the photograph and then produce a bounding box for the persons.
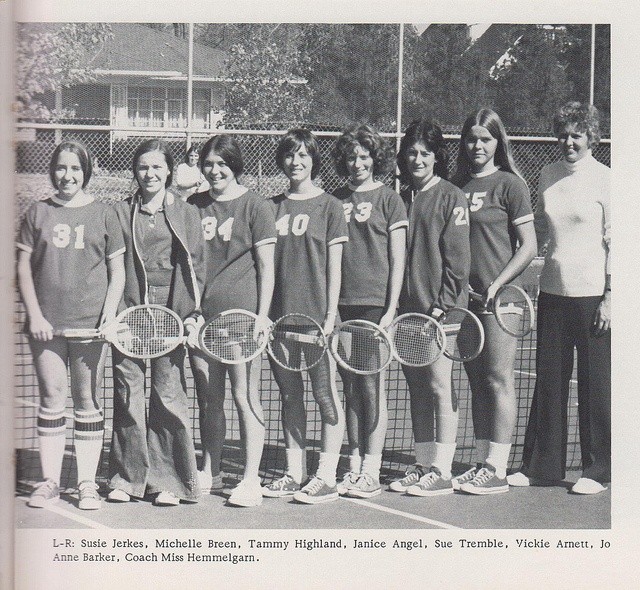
[261,129,349,505]
[332,122,408,497]
[14,140,128,510]
[389,119,470,497]
[186,135,278,507]
[176,146,204,196]
[106,140,208,506]
[506,102,610,495]
[454,108,538,494]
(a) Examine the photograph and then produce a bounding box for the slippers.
[507,470,561,487]
[572,477,609,493]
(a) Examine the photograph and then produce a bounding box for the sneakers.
[293,477,341,503]
[196,470,225,493]
[337,473,358,497]
[230,476,264,505]
[451,463,482,491]
[389,464,425,492]
[107,487,132,500]
[354,473,381,497]
[406,467,453,496]
[29,481,60,507]
[461,463,508,494]
[156,490,180,504]
[78,482,98,509]
[264,475,303,496]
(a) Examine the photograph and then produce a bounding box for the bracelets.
[326,311,336,316]
